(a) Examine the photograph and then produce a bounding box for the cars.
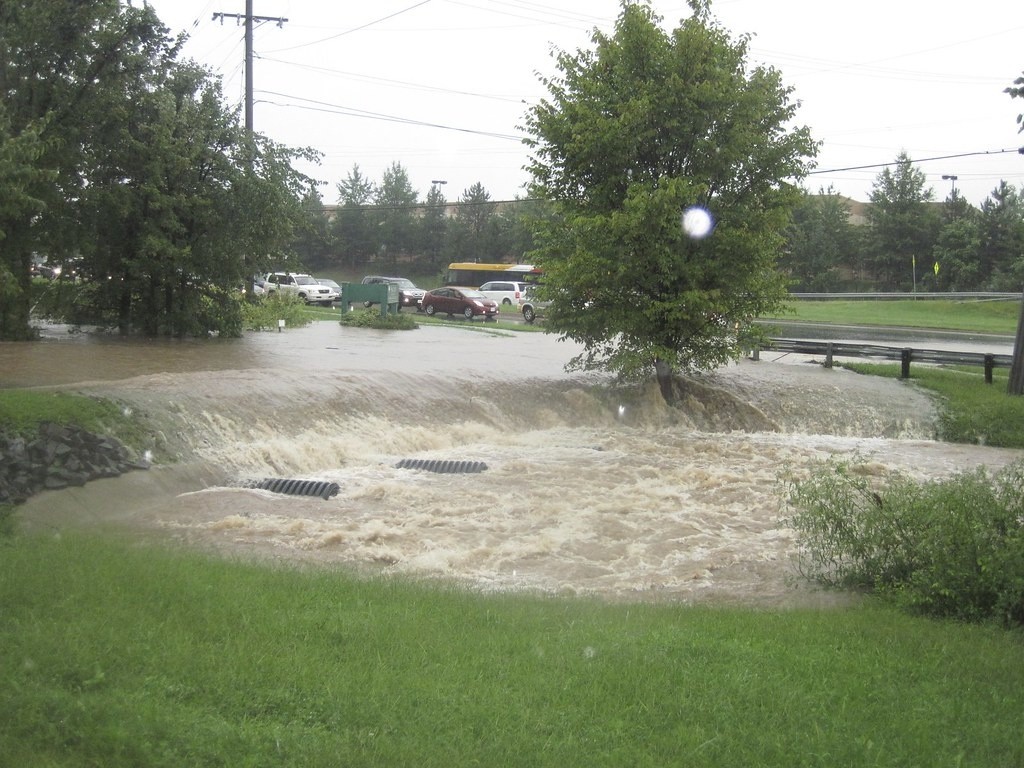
[517,286,590,321]
[239,280,265,294]
[315,279,344,301]
[421,286,500,319]
[30,257,87,281]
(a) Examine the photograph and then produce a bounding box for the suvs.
[476,281,534,306]
[262,273,336,308]
[361,274,429,311]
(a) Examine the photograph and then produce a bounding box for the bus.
[442,262,546,292]
